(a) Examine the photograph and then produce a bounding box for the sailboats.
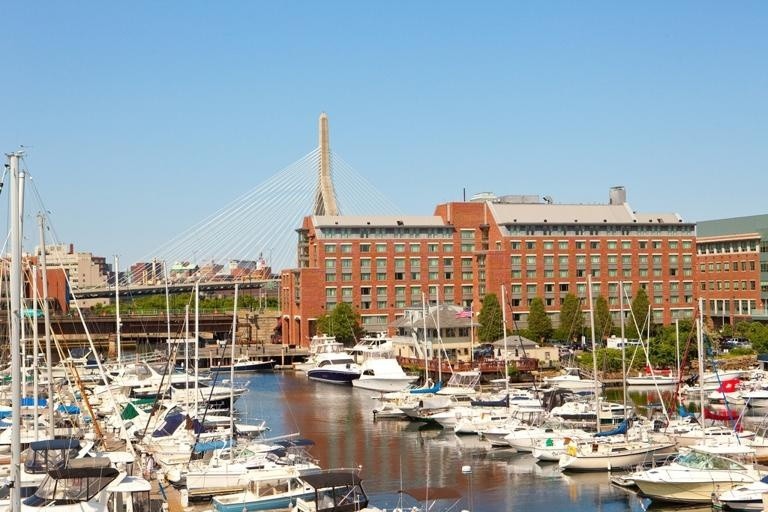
[1,146,367,512]
[356,275,768,512]
